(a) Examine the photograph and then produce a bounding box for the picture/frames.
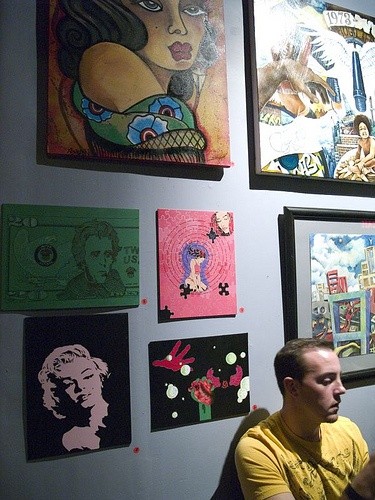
[36,0,236,171]
[245,0,375,186]
[284,204,375,385]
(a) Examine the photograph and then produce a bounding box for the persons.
[236,338,375,500]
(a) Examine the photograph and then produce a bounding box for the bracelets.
[343,483,365,500]
